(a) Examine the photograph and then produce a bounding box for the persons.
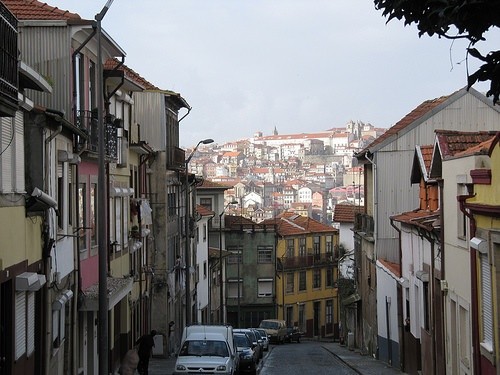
[133,330,157,375]
[168,321,174,338]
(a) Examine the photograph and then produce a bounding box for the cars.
[287,326,302,343]
[232,328,272,367]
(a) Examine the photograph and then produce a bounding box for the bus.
[258,319,289,345]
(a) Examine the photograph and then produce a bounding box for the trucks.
[172,325,240,375]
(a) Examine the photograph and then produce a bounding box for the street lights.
[185,139,215,326]
[220,201,237,324]
